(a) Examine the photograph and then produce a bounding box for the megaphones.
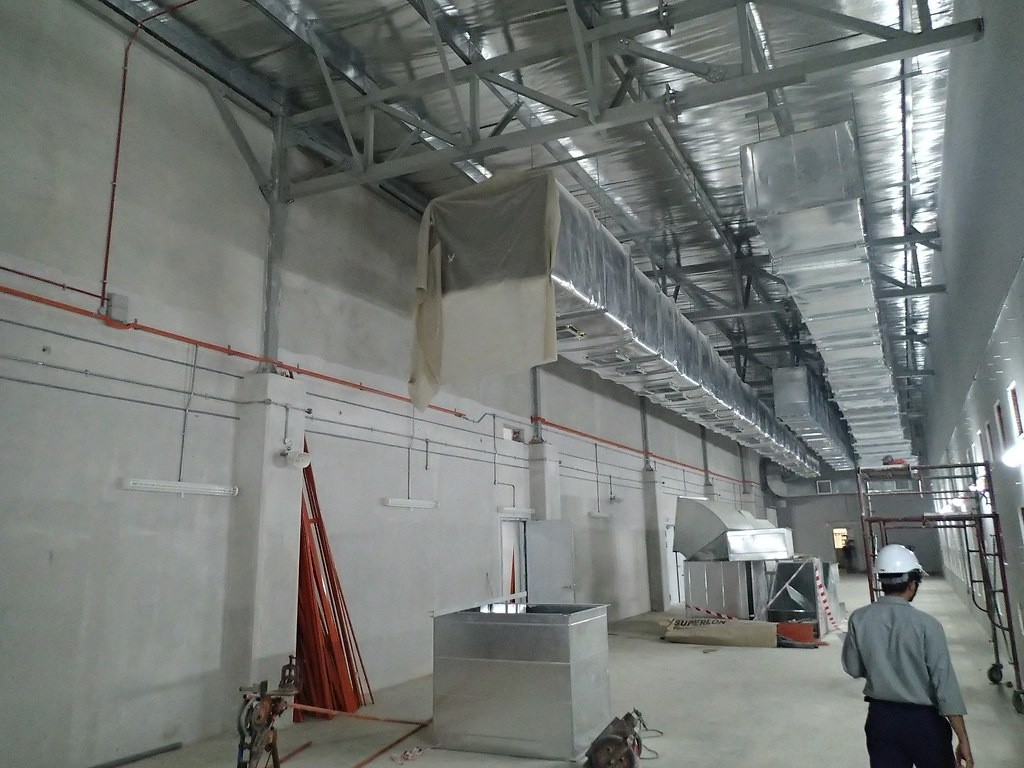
[288,450,312,468]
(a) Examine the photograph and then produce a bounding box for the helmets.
[872,543,922,573]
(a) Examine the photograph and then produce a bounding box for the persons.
[840,544,975,768]
[842,542,853,573]
